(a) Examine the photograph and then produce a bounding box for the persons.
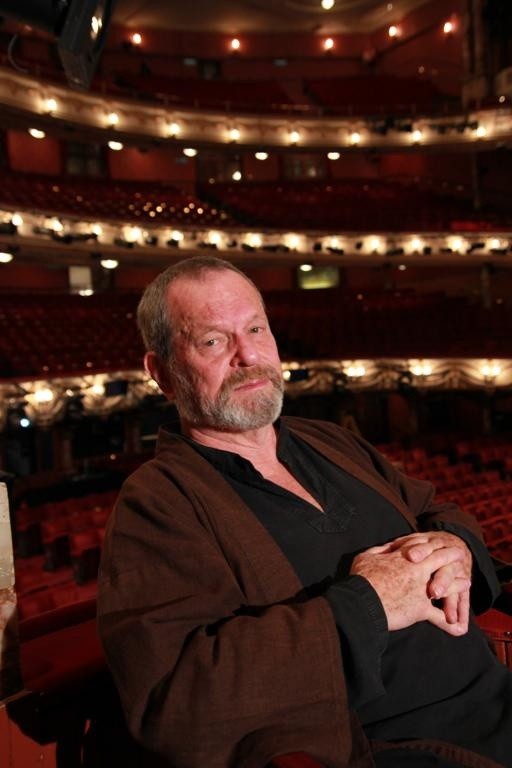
[92,254,512,768]
